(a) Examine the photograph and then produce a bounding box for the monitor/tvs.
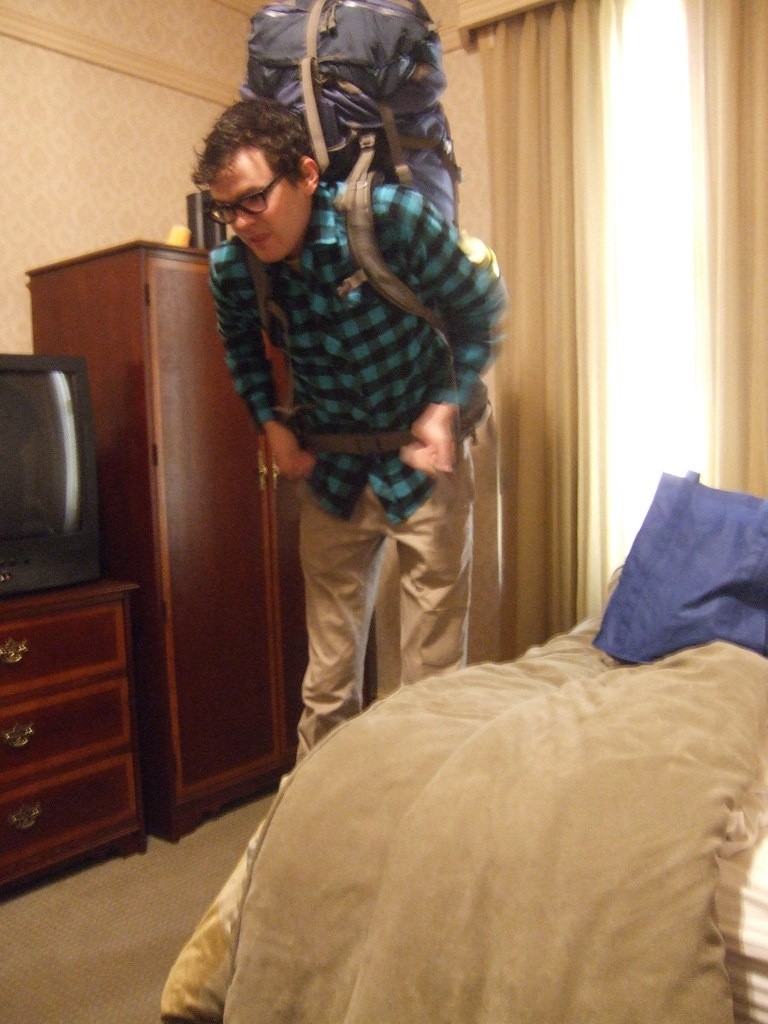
[0,353,102,596]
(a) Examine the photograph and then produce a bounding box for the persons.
[191,100,509,768]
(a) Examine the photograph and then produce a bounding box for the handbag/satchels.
[593,468,768,662]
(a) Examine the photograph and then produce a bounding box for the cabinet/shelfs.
[0,580,147,889]
[25,239,379,845]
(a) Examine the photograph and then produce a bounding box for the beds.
[158,615,768,1024]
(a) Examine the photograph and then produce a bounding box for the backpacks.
[239,0,463,340]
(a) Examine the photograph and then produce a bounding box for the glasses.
[205,165,294,226]
